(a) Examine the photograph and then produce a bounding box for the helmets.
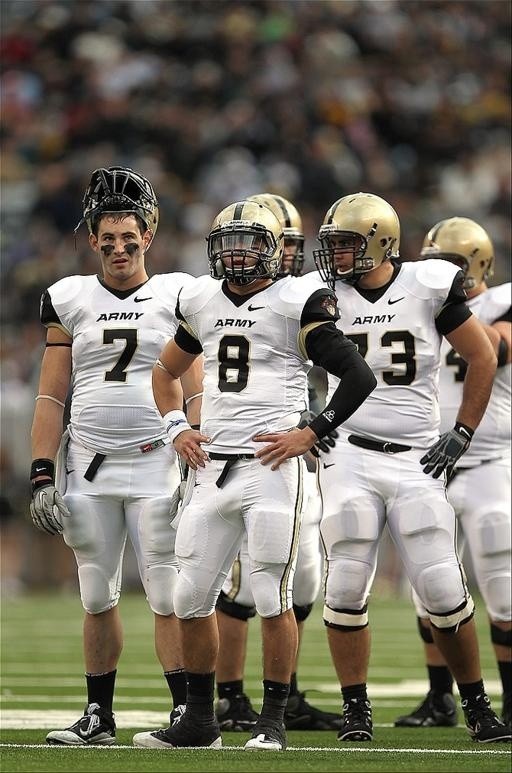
[72,166,160,253]
[234,194,307,275]
[417,217,496,298]
[315,192,400,283]
[206,200,285,282]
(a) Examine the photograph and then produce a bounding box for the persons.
[133,202,374,751]
[394,217,512,729]
[215,194,345,730]
[0,0,512,535]
[299,194,512,743]
[30,167,204,744]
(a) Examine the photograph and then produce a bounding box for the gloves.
[29,479,71,537]
[294,409,340,458]
[420,423,474,490]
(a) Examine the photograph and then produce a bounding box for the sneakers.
[244,715,287,752]
[133,704,223,748]
[282,693,343,731]
[461,693,510,743]
[216,695,262,732]
[395,689,459,726]
[45,702,117,745]
[338,696,374,741]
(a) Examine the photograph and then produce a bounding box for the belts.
[210,451,254,487]
[348,434,410,454]
